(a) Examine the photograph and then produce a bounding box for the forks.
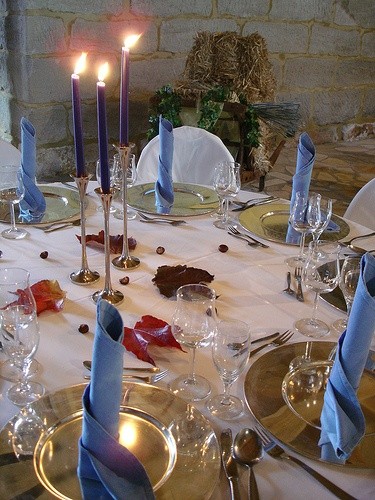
[337,232,375,247]
[137,211,175,222]
[32,221,74,230]
[252,425,358,500]
[249,330,296,358]
[228,226,269,249]
[293,266,306,302]
[85,370,168,384]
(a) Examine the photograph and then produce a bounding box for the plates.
[242,342,375,469]
[119,182,225,217]
[0,381,221,500]
[238,203,350,246]
[0,186,89,224]
[312,258,362,315]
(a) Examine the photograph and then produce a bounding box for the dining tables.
[0,178,375,500]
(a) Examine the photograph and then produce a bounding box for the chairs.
[135,125,235,190]
[0,138,36,188]
[343,178,374,231]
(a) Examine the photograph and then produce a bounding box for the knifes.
[343,253,362,258]
[220,427,241,500]
[231,197,280,211]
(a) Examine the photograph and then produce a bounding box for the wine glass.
[282,318,332,409]
[214,160,241,230]
[294,240,342,338]
[112,153,136,220]
[285,190,321,271]
[0,267,37,356]
[6,381,55,466]
[166,373,217,471]
[0,165,27,240]
[96,159,118,214]
[168,284,216,400]
[1,305,45,406]
[206,318,251,421]
[332,256,365,333]
[211,163,236,219]
[303,195,332,259]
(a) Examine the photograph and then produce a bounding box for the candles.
[96,62,110,193]
[120,34,144,146]
[71,52,90,178]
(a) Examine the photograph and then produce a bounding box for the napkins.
[155,117,174,215]
[16,117,45,221]
[319,252,375,465]
[285,133,316,245]
[77,299,156,499]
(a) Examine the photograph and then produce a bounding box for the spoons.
[228,231,258,248]
[83,360,160,374]
[226,331,280,351]
[232,427,264,500]
[232,195,274,206]
[348,244,375,255]
[282,271,295,296]
[43,218,86,233]
[140,219,185,226]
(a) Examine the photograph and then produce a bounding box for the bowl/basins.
[308,239,341,256]
